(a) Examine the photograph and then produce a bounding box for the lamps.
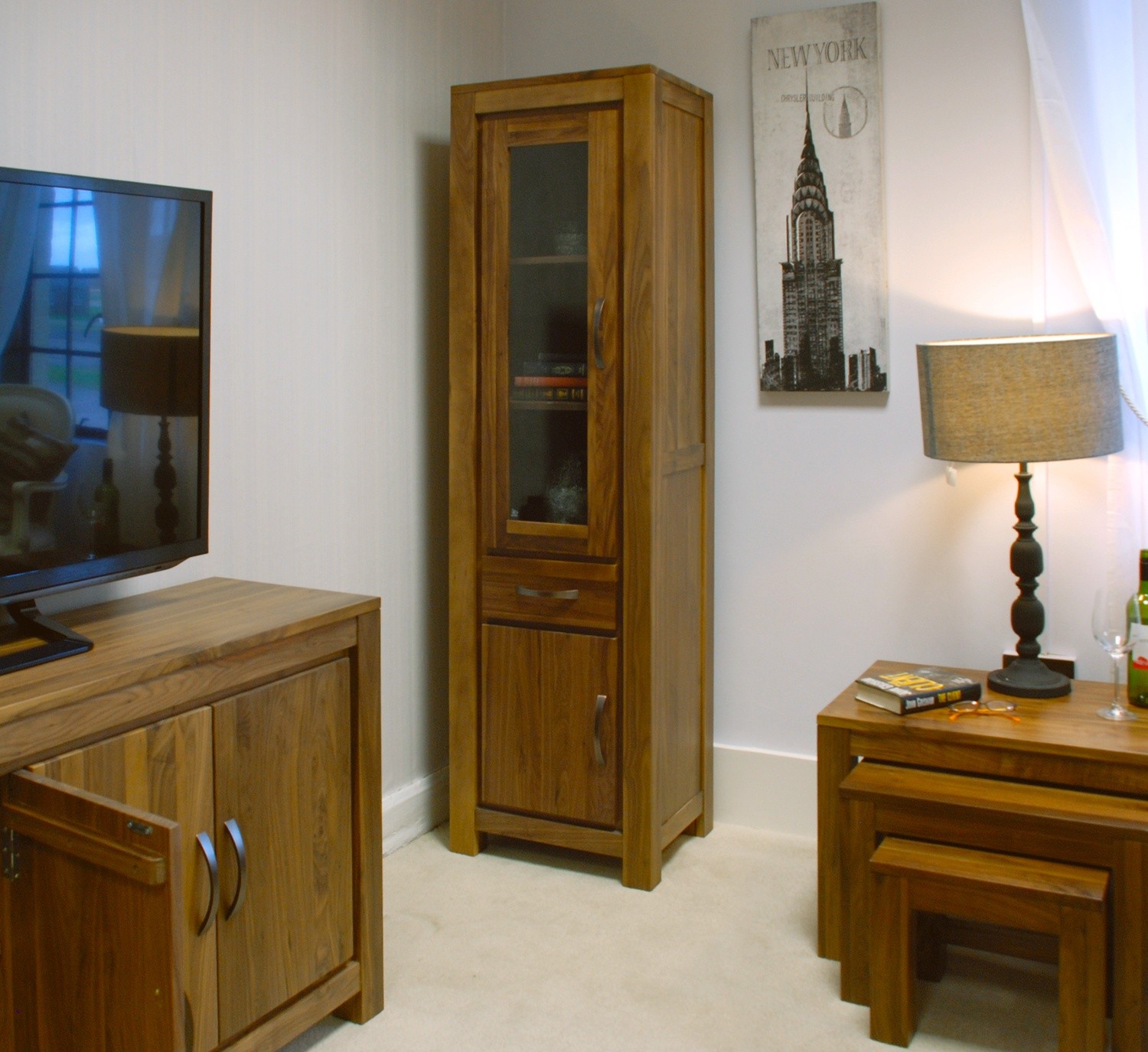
[918,330,1123,698]
[96,325,200,544]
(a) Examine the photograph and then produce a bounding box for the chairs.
[0,383,72,570]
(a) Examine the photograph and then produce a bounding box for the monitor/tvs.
[0,166,211,675]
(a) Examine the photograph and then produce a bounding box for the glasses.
[947,699,1022,724]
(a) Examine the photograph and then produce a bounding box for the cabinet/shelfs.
[0,572,389,1052]
[441,65,720,890]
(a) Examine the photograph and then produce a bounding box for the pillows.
[0,410,82,481]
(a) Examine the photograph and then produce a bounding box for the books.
[854,666,982,716]
[510,361,588,401]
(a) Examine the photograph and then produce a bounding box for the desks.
[814,657,1148,1052]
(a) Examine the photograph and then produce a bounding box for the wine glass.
[1090,590,1142,721]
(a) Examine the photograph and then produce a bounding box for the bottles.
[1125,550,1148,710]
